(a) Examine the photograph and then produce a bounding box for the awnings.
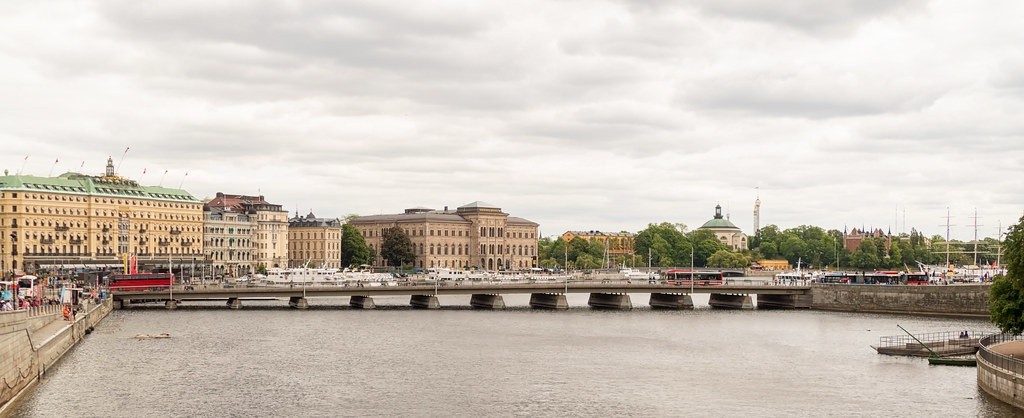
[40,263,126,270]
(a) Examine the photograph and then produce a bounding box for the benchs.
[948,339,979,348]
[905,341,944,351]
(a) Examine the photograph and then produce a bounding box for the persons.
[0,283,107,320]
[182,278,363,291]
[627,275,807,286]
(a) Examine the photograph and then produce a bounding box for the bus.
[661,269,723,287]
[902,271,930,285]
[102,272,175,290]
[821,273,900,286]
[773,273,812,287]
